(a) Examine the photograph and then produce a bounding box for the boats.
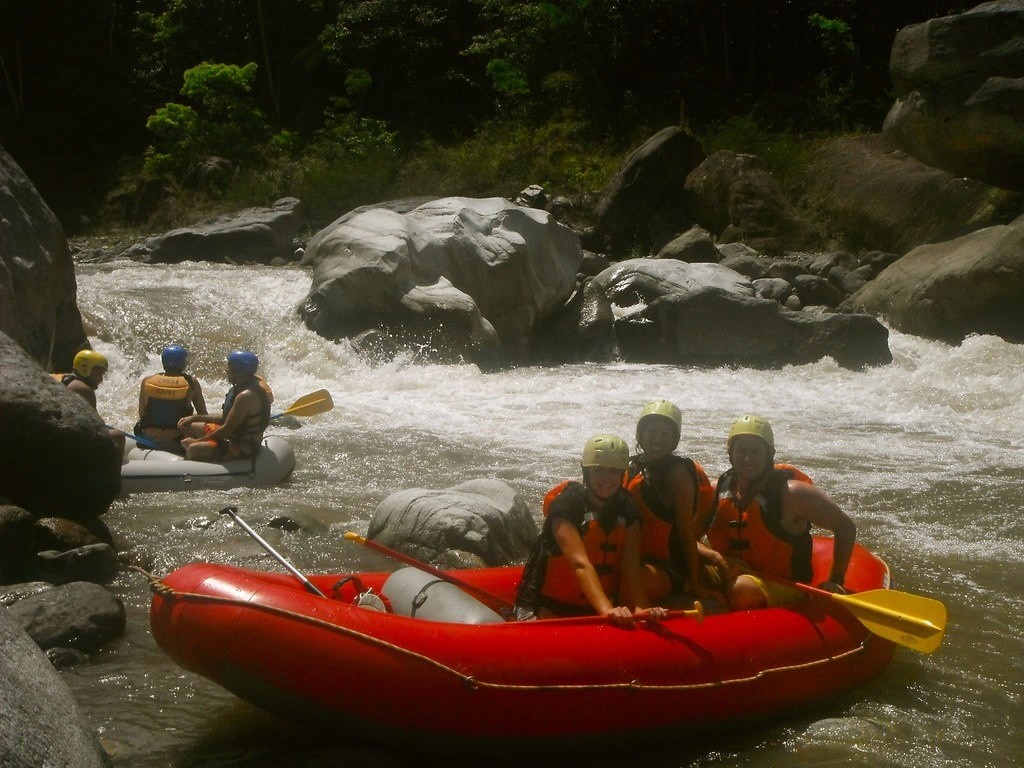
[118,434,296,495]
[149,532,897,758]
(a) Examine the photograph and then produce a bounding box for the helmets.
[73,350,109,378]
[582,433,629,471]
[161,345,187,372]
[637,399,682,450]
[726,415,774,457]
[227,351,259,375]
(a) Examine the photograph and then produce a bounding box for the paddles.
[269,389,334,421]
[104,423,165,451]
[728,558,947,654]
[503,601,705,623]
[344,531,513,613]
[220,506,327,598]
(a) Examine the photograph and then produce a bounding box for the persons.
[177,348,275,459]
[619,400,720,617]
[512,432,668,629]
[704,413,857,610]
[133,345,211,453]
[48,350,124,464]
